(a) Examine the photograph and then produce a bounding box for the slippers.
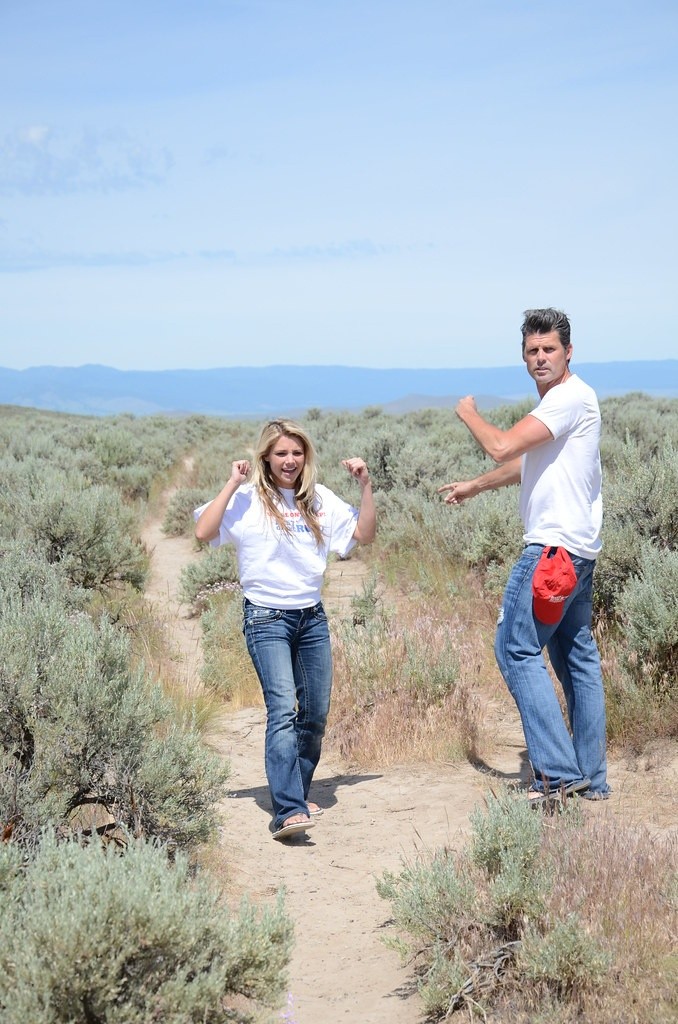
[581,791,608,800]
[310,808,323,816]
[523,778,592,805]
[272,813,315,839]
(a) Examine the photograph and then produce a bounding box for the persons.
[193,421,376,838]
[438,309,613,804]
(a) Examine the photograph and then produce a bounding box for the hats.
[532,545,577,625]
